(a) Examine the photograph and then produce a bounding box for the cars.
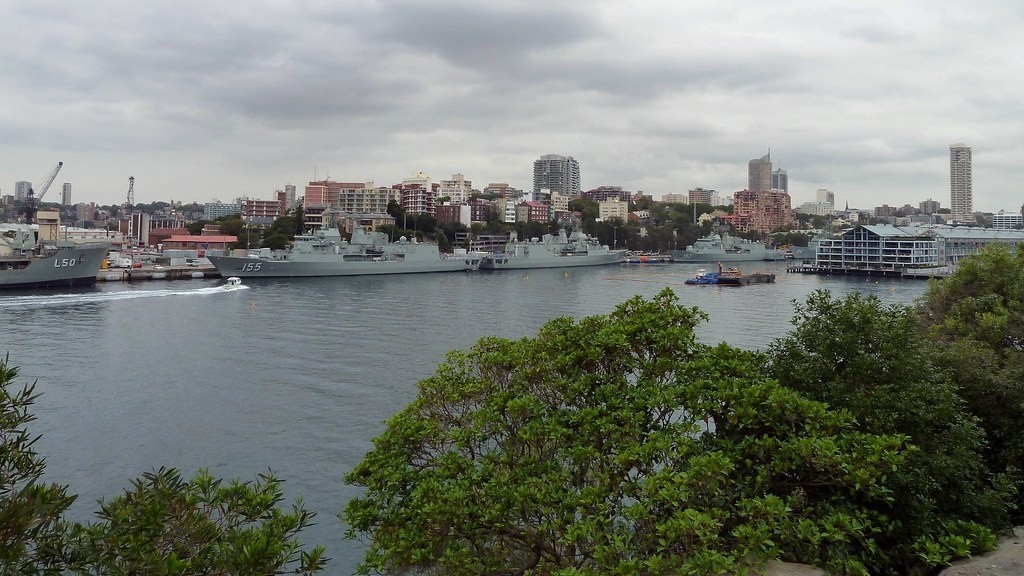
[153,265,163,269]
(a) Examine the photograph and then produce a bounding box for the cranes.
[127,176,134,208]
[19,161,64,224]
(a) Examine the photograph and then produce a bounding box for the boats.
[205,207,492,279]
[788,237,832,258]
[669,221,787,262]
[0,227,112,289]
[684,262,776,285]
[479,215,628,269]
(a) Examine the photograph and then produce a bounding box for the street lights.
[614,226,618,248]
[547,225,551,233]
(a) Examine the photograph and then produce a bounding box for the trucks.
[111,259,132,267]
[186,259,199,267]
